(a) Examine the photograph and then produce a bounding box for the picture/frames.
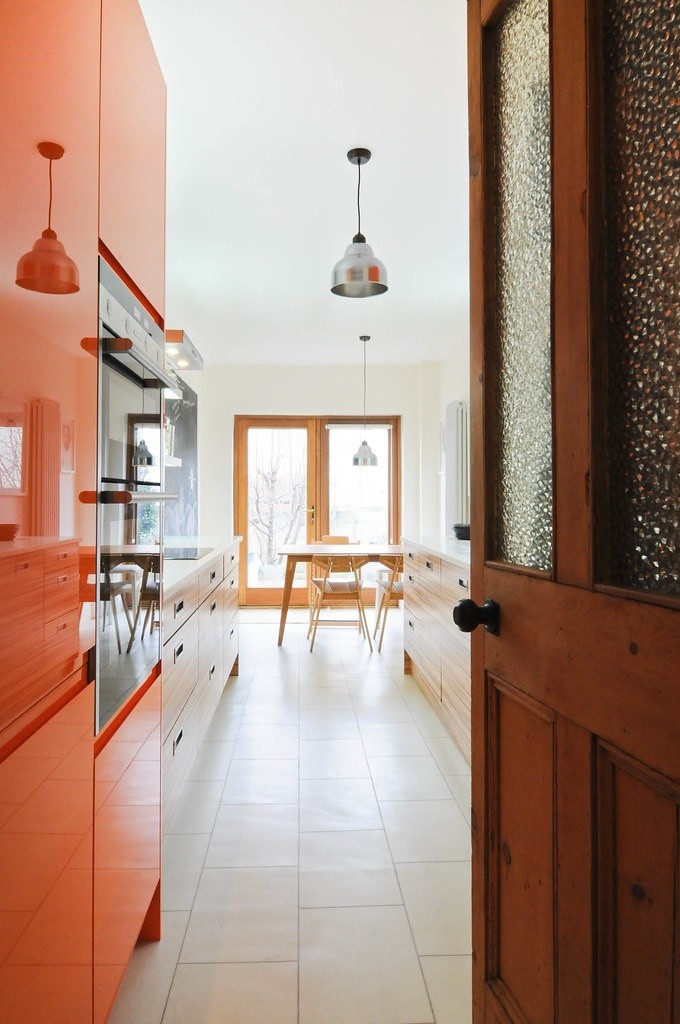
[60,413,77,474]
[0,391,31,496]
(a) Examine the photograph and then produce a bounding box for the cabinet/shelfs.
[162,535,243,842]
[0,534,84,732]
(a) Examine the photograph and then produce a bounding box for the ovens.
[95,253,162,735]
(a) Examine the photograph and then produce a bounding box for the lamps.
[15,157,80,295]
[330,162,388,298]
[131,367,154,467]
[352,341,378,467]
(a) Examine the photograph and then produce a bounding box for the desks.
[79,545,159,562]
[278,543,403,647]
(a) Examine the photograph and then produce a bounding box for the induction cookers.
[164,547,215,560]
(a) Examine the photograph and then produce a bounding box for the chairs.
[79,555,135,655]
[126,554,159,655]
[306,553,373,652]
[310,536,361,632]
[373,554,403,653]
